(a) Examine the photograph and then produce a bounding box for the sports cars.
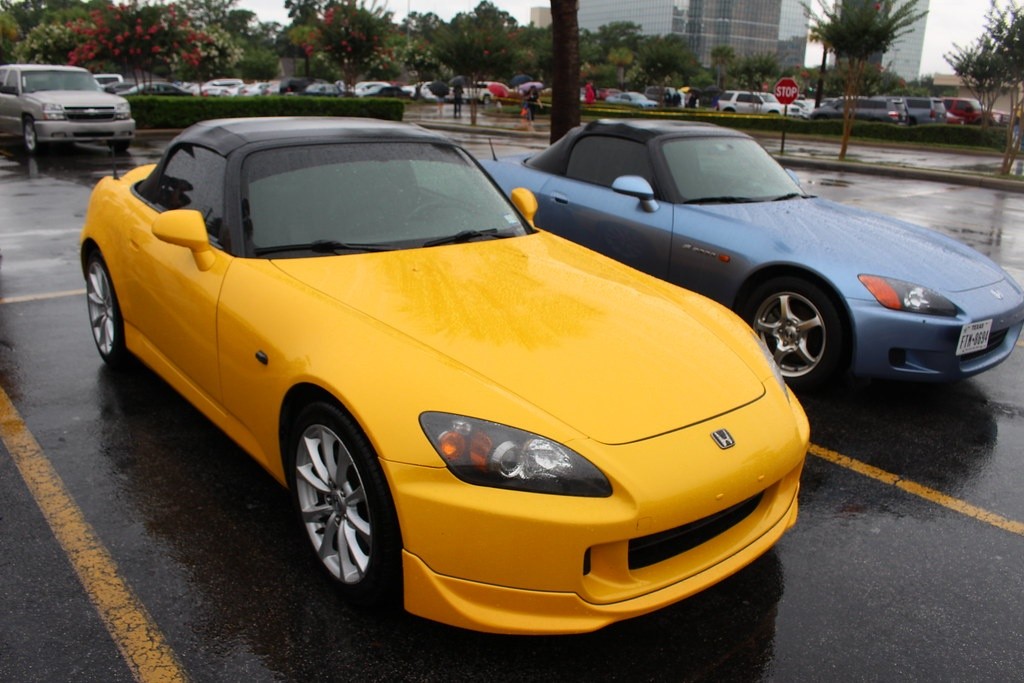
[80,113,812,637]
[471,119,1024,395]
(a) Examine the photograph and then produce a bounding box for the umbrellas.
[509,74,533,85]
[704,86,723,95]
[429,81,450,96]
[691,87,702,93]
[680,87,690,93]
[519,81,544,91]
[487,82,509,97]
[450,76,467,85]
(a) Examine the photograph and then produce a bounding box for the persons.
[662,88,672,107]
[689,93,698,108]
[711,92,721,111]
[671,88,682,107]
[436,95,448,118]
[685,91,692,107]
[584,80,597,103]
[524,86,543,122]
[453,85,464,118]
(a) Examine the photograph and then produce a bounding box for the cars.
[115,82,192,98]
[352,81,453,100]
[462,83,496,103]
[607,90,659,109]
[201,78,339,98]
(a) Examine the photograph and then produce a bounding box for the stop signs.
[775,78,799,104]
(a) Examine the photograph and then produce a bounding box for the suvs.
[716,89,802,117]
[942,98,983,125]
[0,64,135,155]
[904,97,947,126]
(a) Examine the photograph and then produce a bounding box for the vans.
[809,96,909,125]
[646,85,676,102]
